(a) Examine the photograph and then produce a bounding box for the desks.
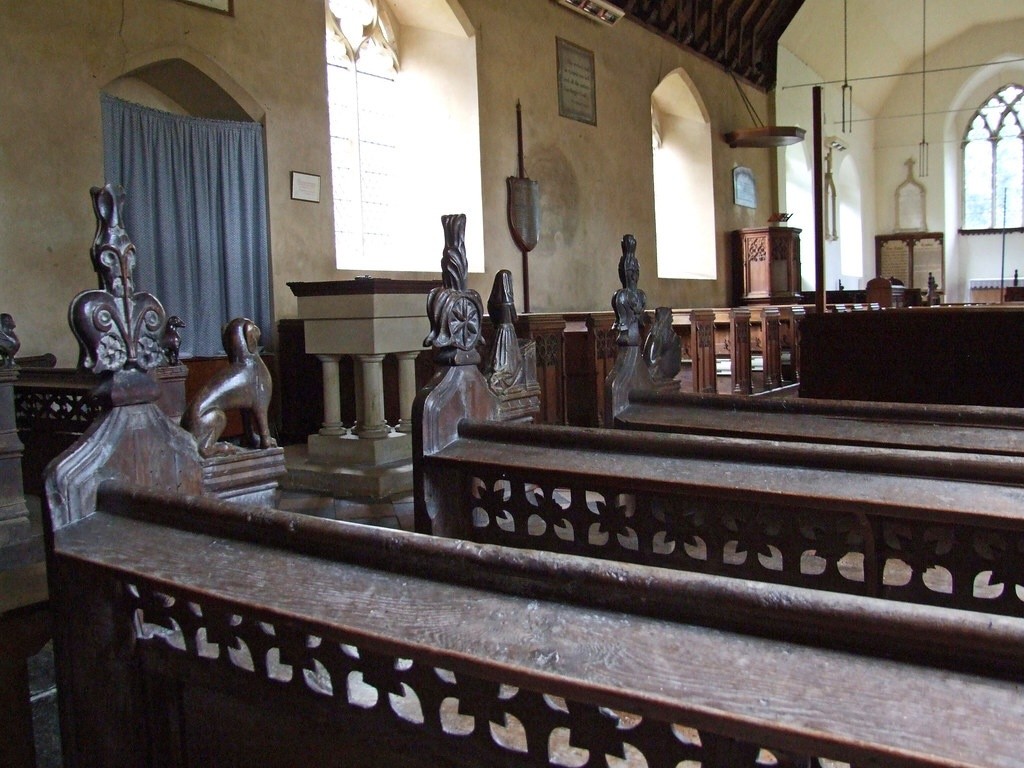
[41,183,1024,767]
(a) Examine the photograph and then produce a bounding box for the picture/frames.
[289,170,320,204]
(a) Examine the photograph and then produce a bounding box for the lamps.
[824,135,849,151]
[557,0,624,28]
